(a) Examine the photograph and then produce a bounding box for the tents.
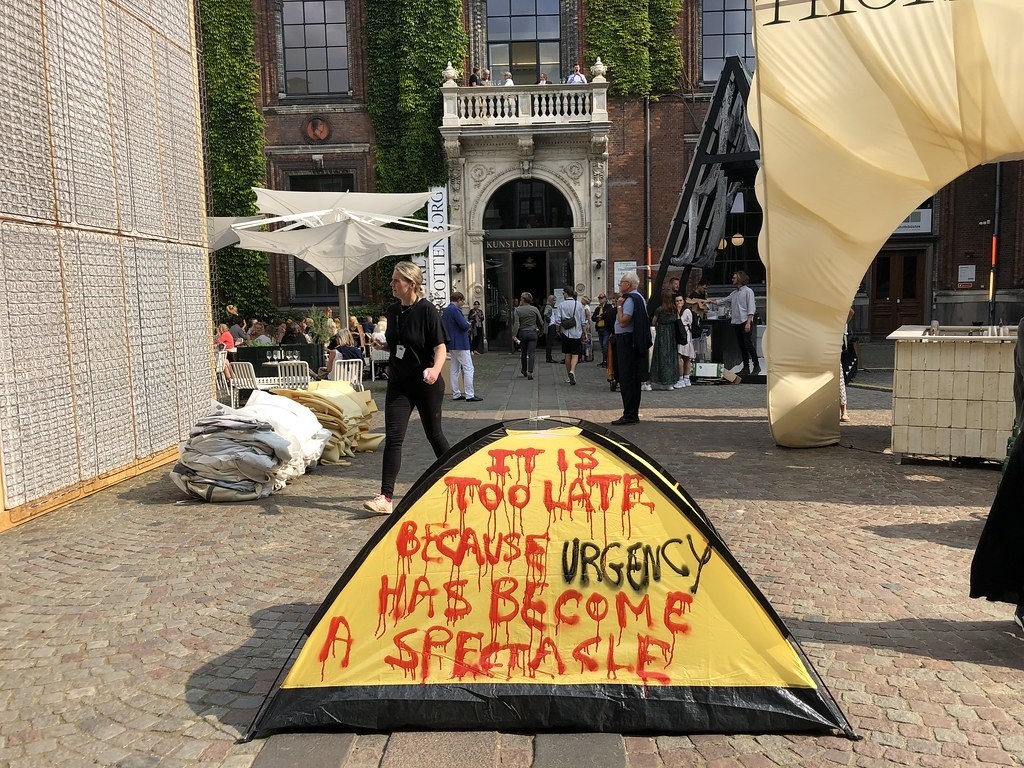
[748,0,1024,446]
[240,417,862,740]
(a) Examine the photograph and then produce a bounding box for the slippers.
[840,414,851,422]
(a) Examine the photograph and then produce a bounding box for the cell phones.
[368,333,381,346]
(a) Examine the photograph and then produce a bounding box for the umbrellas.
[207,186,462,329]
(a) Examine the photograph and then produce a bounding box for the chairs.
[335,359,363,392]
[219,342,227,351]
[229,362,279,410]
[356,325,371,357]
[214,351,230,398]
[278,361,310,391]
[371,340,390,382]
[234,338,243,346]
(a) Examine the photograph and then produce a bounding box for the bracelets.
[617,306,622,309]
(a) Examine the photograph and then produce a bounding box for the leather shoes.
[466,395,483,402]
[453,396,465,400]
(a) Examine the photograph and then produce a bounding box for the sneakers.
[683,379,691,387]
[641,382,652,391]
[363,492,393,514]
[1013,607,1024,632]
[565,371,576,385]
[611,415,641,425]
[375,372,389,379]
[674,381,686,389]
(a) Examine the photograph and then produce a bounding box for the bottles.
[272,350,277,362]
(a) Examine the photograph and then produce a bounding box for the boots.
[751,361,761,375]
[735,362,750,374]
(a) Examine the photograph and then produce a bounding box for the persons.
[647,278,710,390]
[210,304,388,382]
[839,306,859,423]
[513,287,621,386]
[700,272,761,376]
[442,292,484,402]
[469,64,587,87]
[610,273,653,425]
[441,301,484,359]
[363,262,450,515]
[969,319,1024,630]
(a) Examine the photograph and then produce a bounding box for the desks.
[214,361,221,390]
[262,361,303,388]
[226,347,237,352]
[702,314,758,370]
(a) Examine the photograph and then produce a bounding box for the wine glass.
[266,351,272,363]
[285,351,293,361]
[292,350,300,361]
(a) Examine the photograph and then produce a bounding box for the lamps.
[593,258,606,270]
[312,154,324,170]
[731,213,744,246]
[718,238,727,249]
[522,256,536,269]
[451,264,465,272]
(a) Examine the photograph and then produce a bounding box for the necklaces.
[401,295,419,313]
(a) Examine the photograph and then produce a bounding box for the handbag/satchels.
[561,301,577,330]
[687,308,703,339]
[675,319,688,346]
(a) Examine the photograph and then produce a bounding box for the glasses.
[563,293,565,295]
[617,280,628,284]
[598,296,605,299]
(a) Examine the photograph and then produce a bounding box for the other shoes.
[653,383,674,391]
[446,353,451,360]
[597,361,606,368]
[546,359,559,363]
[521,369,527,377]
[560,358,565,364]
[528,371,533,380]
